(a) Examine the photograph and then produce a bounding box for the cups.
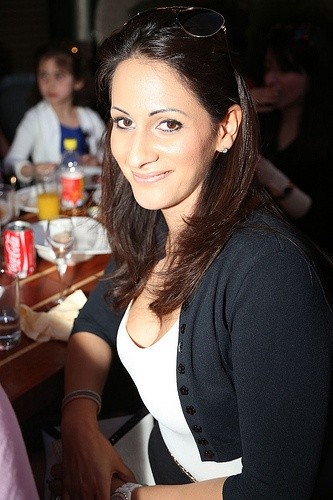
[0,270,21,351]
[31,170,60,220]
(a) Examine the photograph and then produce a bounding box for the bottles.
[57,139,84,210]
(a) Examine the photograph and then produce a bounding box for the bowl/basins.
[30,216,114,267]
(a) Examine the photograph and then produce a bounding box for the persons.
[0,384,39,500]
[252,19,333,303]
[3,48,106,183]
[49,6,333,500]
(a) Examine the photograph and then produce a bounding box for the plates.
[14,183,89,212]
[55,165,103,188]
[91,188,102,205]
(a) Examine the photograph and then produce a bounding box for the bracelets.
[62,389,102,414]
[273,179,294,202]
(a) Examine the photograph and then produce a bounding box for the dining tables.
[0,189,115,402]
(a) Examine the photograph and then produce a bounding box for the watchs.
[111,481,142,500]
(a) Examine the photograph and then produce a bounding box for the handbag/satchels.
[36,409,157,500]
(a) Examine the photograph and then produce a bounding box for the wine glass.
[0,183,14,269]
[45,215,77,304]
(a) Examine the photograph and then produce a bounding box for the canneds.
[4,220,36,278]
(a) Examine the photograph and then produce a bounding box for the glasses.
[122,6,233,72]
